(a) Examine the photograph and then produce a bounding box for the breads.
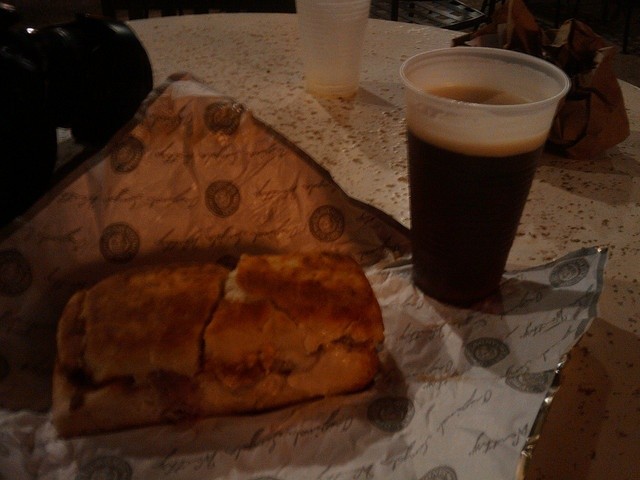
[52,250,385,438]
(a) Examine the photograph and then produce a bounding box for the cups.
[295,0,370,97]
[399,46,570,306]
